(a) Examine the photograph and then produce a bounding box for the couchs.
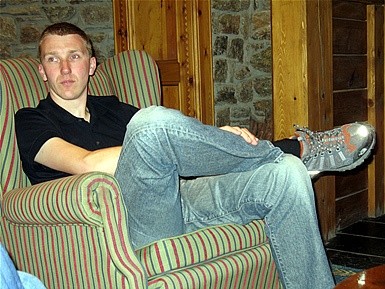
[0,49,282,289]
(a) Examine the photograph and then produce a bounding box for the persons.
[14,22,376,289]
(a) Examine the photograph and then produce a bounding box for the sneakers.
[288,120,377,180]
